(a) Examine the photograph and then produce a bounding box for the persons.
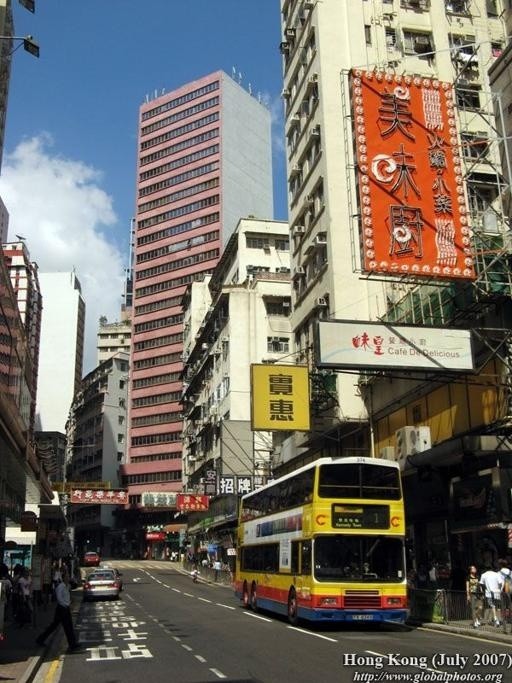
[466,559,512,629]
[34,573,86,654]
[172,551,234,584]
[359,560,371,579]
[406,569,421,624]
[41,557,81,612]
[0,561,41,639]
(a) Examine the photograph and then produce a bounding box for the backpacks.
[500,570,512,592]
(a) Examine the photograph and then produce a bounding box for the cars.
[82,566,123,600]
[84,551,100,566]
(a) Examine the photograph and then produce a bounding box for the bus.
[234,456,410,627]
